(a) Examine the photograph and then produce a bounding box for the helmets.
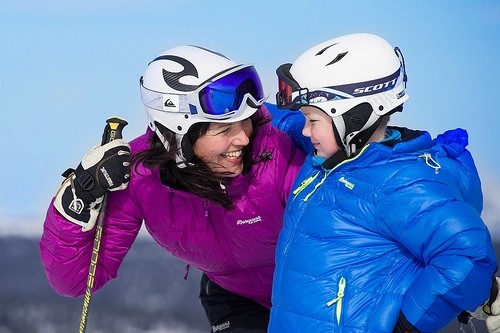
[139,44,266,169]
[274,31,409,158]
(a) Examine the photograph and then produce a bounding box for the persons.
[255,29,499,332]
[37,44,306,332]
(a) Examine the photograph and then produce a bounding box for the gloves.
[52,139,133,234]
